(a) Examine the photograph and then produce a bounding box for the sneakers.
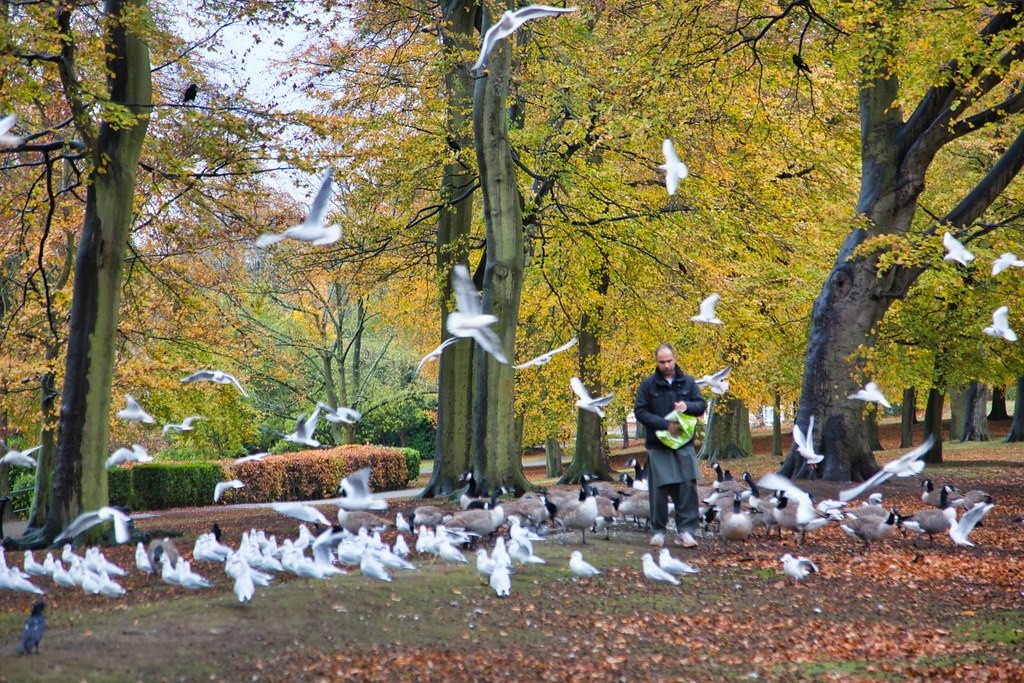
[673,533,698,548]
[650,534,665,546]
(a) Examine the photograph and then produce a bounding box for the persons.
[632,345,708,548]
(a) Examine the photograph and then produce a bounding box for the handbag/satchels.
[657,410,698,450]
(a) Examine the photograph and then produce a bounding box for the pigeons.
[780,552,818,588]
[256,174,343,253]
[469,5,580,72]
[279,407,324,447]
[658,547,700,575]
[694,366,732,395]
[689,293,725,325]
[180,370,249,398]
[981,305,1018,341]
[329,468,391,510]
[838,432,941,502]
[640,553,681,586]
[943,231,975,268]
[947,503,995,548]
[414,337,458,377]
[792,415,825,470]
[991,252,1024,276]
[272,501,332,526]
[0,445,47,469]
[161,417,201,436]
[565,551,603,587]
[511,336,578,369]
[233,452,269,465]
[105,444,154,469]
[446,264,508,367]
[847,381,893,409]
[318,401,361,424]
[54,506,133,543]
[0,512,546,603]
[214,478,246,503]
[657,139,688,197]
[570,377,614,419]
[113,394,156,424]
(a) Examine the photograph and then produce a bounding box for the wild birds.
[793,53,812,73]
[212,522,221,542]
[15,602,49,656]
[0,115,24,147]
[330,458,995,551]
[445,136,461,155]
[183,84,198,107]
[677,261,687,274]
